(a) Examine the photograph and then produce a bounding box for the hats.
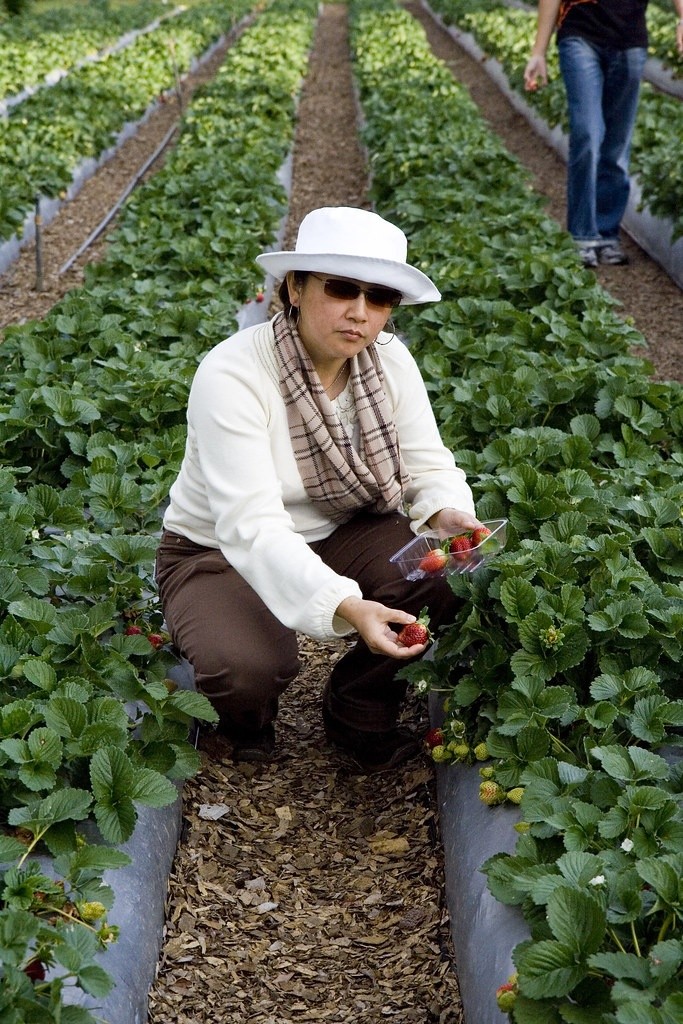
[256,207,442,306]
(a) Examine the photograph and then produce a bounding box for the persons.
[155,206,492,773]
[522,0,683,266]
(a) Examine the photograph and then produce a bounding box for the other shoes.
[233,724,275,762]
[322,701,419,772]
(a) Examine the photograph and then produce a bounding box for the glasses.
[310,273,405,308]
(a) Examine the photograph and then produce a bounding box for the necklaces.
[325,362,348,391]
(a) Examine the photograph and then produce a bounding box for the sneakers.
[598,246,628,265]
[579,247,598,266]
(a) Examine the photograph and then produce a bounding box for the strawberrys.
[126,625,141,635]
[397,622,435,647]
[425,727,444,748]
[148,634,163,650]
[418,527,492,571]
